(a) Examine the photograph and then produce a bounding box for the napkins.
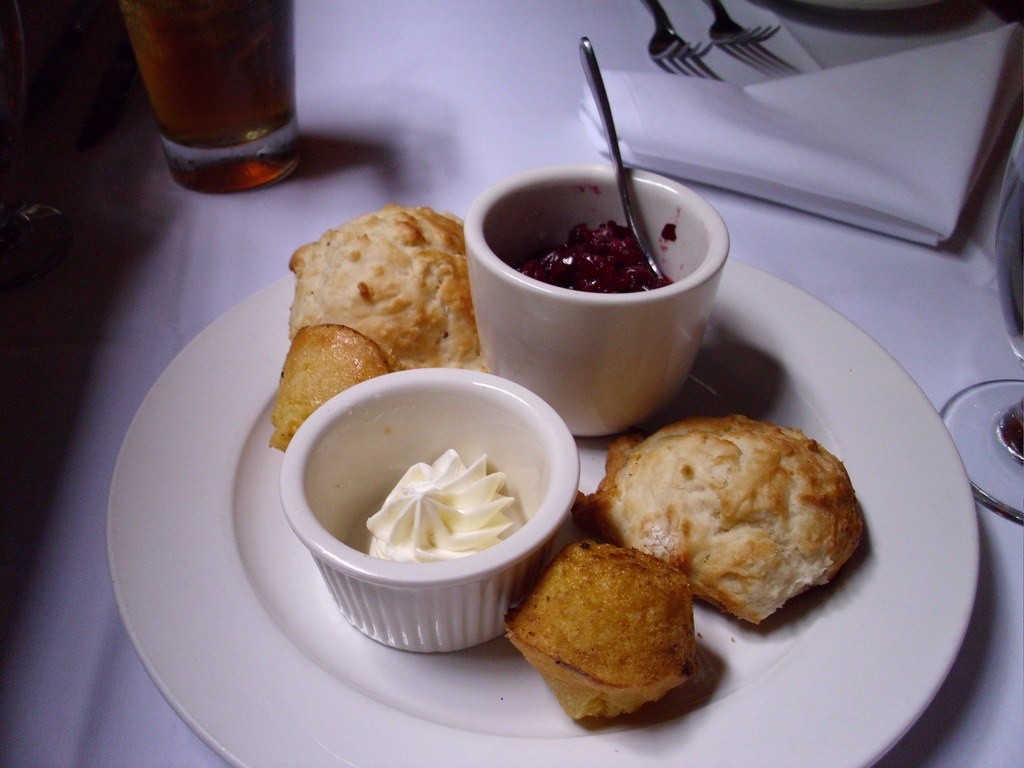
[579,22,1024,249]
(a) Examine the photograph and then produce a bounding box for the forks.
[642,0,713,60]
[703,0,781,47]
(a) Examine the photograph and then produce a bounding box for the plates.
[106,257,978,768]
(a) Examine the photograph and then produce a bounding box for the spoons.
[579,35,666,281]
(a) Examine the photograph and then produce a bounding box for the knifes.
[78,36,137,151]
[23,0,103,127]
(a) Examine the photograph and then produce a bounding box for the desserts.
[278,368,581,655]
[269,202,479,453]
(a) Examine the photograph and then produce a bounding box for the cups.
[465,163,728,434]
[119,0,301,193]
[280,365,582,655]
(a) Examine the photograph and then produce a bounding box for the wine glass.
[0,0,72,290]
[940,112,1024,525]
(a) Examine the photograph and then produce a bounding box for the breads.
[502,538,701,720]
[569,413,865,624]
